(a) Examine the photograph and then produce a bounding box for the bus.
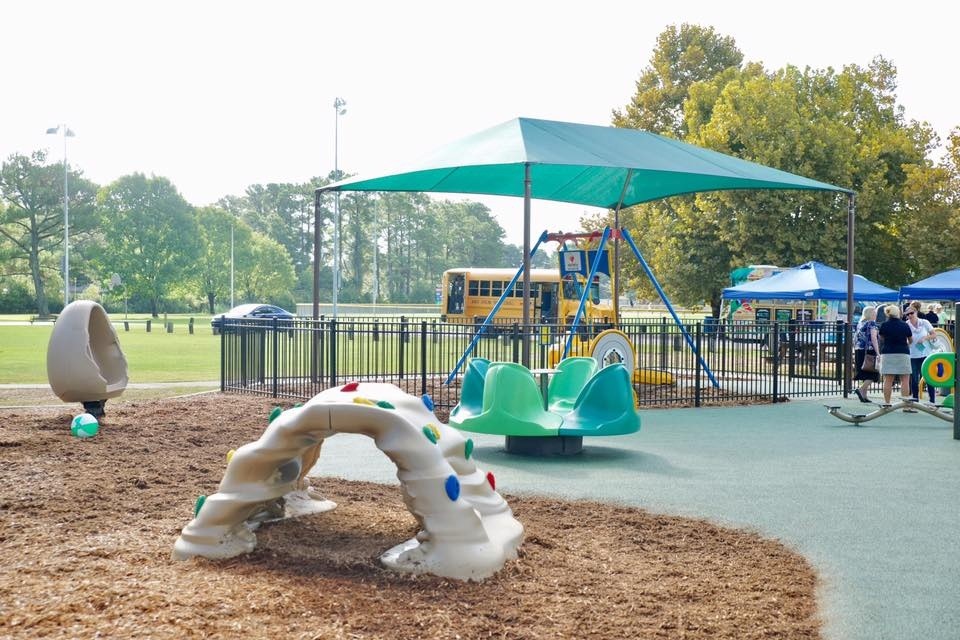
[440,267,622,342]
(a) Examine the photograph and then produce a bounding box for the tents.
[715,261,900,376]
[310,117,857,399]
[899,268,960,313]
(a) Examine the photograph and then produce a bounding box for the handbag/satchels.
[861,356,879,372]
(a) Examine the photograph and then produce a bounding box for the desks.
[777,342,837,369]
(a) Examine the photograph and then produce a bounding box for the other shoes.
[903,408,917,413]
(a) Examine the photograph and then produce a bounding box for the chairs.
[450,355,642,436]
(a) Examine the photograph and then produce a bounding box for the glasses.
[905,311,915,316]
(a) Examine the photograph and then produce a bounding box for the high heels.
[864,397,872,403]
[853,389,865,402]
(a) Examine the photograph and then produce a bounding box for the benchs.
[30,315,57,325]
[761,355,800,360]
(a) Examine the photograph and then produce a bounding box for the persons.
[854,302,950,413]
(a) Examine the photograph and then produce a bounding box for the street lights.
[332,95,348,320]
[45,124,76,306]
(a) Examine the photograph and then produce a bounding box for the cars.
[211,303,296,338]
